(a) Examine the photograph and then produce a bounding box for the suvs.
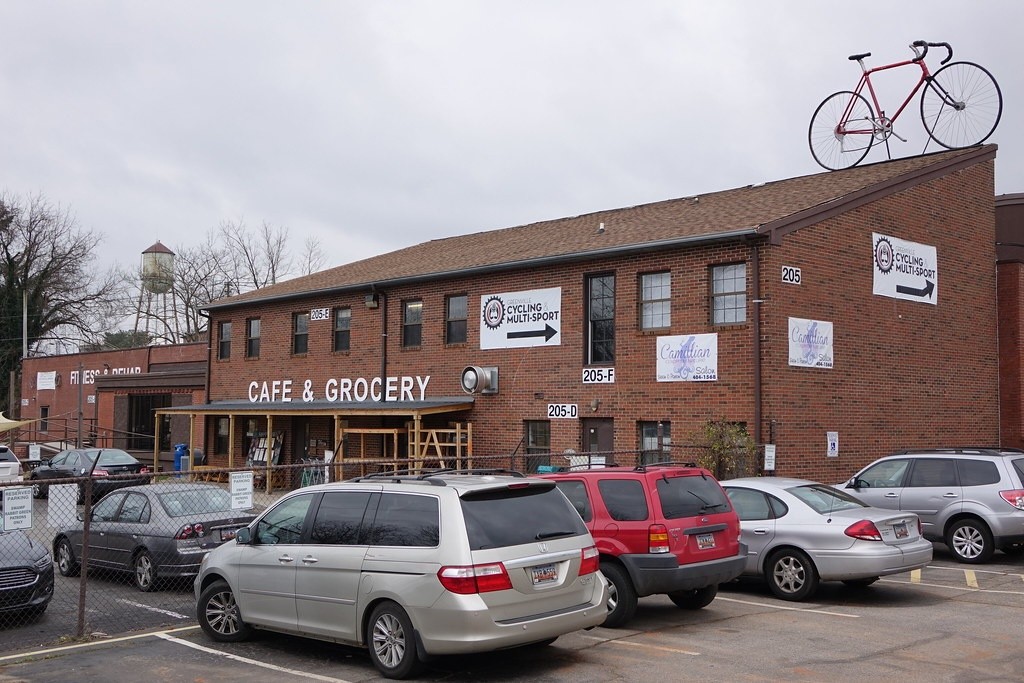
[829,445,1024,565]
[525,460,748,630]
[193,469,611,680]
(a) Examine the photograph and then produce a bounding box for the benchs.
[193,466,229,483]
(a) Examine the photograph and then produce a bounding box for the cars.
[721,475,934,602]
[51,482,271,592]
[0,445,25,501]
[0,510,55,616]
[28,448,151,506]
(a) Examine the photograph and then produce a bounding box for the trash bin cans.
[174,444,188,478]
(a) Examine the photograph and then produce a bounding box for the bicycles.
[808,39,1004,173]
